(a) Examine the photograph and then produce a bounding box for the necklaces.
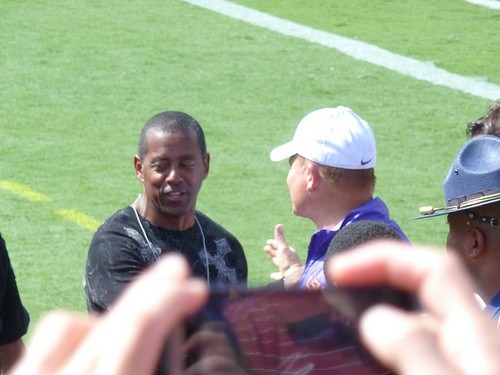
[133,194,210,289]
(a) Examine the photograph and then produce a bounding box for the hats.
[413,135,500,220]
[270,106,377,169]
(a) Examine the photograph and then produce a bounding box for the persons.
[322,219,405,309]
[415,135,500,326]
[0,232,500,375]
[85,110,248,316]
[263,106,411,291]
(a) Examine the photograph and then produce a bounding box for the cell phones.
[187,276,418,375]
